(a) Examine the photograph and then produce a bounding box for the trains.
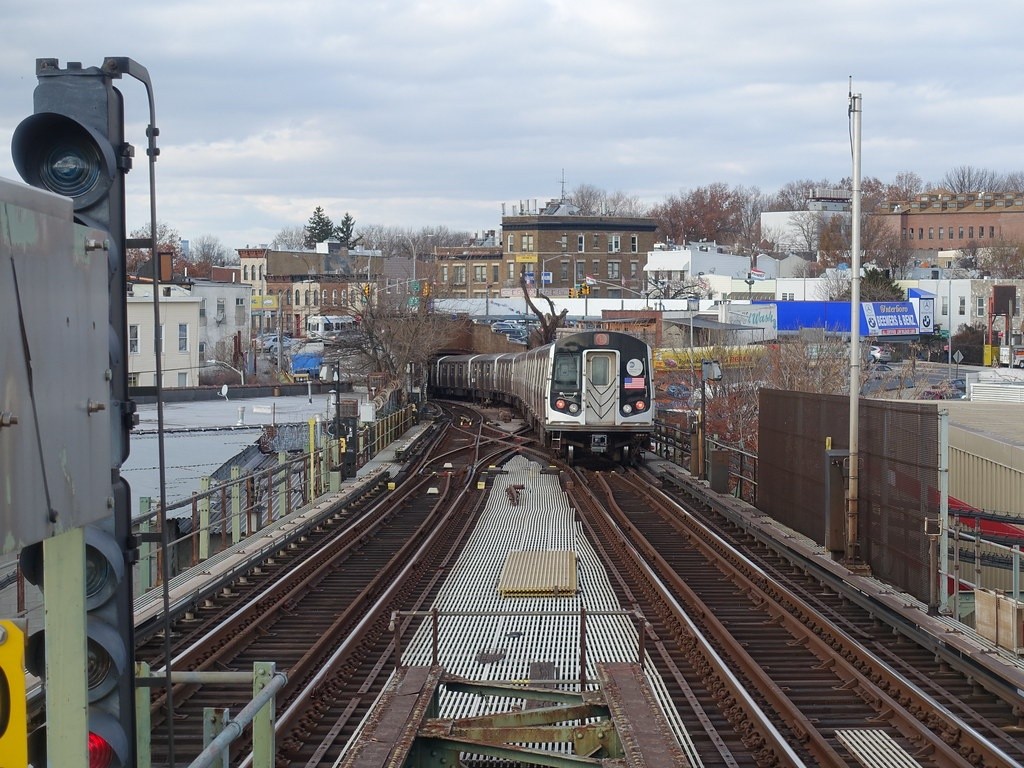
[426,331,657,470]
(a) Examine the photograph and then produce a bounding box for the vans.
[868,345,892,364]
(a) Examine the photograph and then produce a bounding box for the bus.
[307,316,364,348]
[999,329,1024,369]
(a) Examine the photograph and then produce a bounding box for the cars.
[251,333,293,353]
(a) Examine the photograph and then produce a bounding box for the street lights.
[395,234,434,281]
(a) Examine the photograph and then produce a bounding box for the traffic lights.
[13,112,115,212]
[19,525,139,768]
